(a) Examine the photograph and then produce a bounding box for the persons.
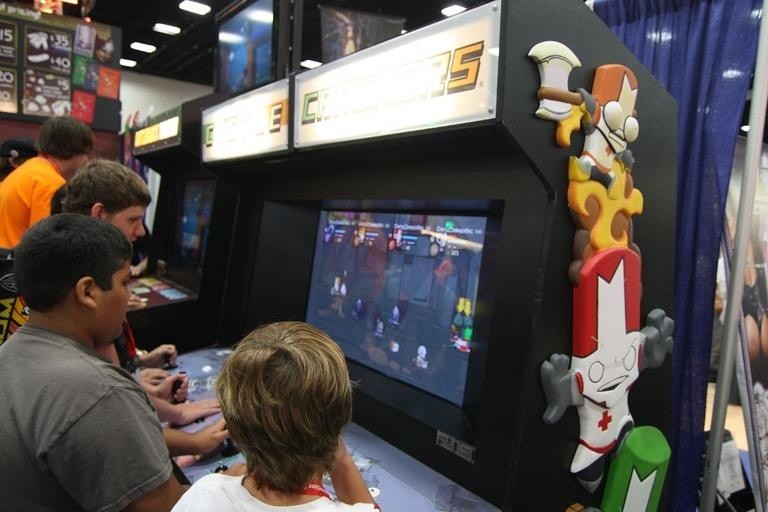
[170,320,381,512]
[1,116,247,511]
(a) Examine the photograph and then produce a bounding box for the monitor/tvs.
[302,200,508,412]
[169,175,220,267]
[300,0,407,74]
[214,1,292,98]
